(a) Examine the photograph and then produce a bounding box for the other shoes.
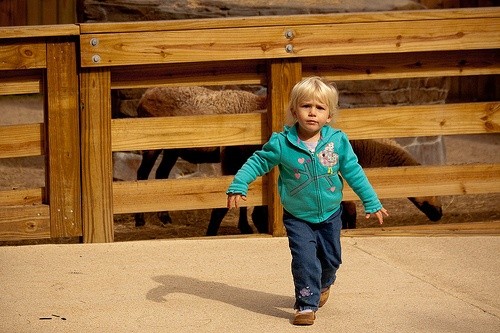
[293,309,315,325]
[319,287,330,307]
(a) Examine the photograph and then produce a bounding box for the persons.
[224,74,389,325]
